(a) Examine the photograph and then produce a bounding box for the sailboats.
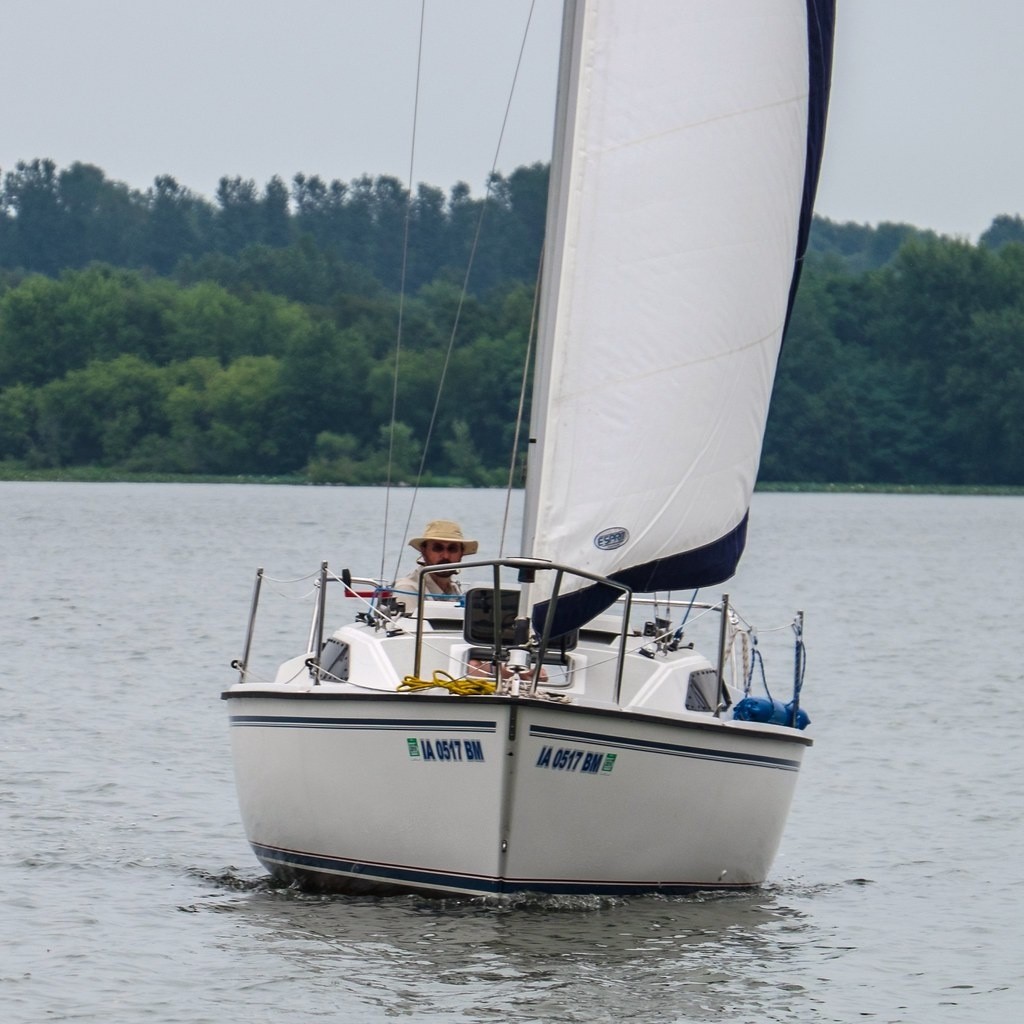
[218,0,840,904]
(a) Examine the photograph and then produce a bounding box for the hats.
[408,519,478,555]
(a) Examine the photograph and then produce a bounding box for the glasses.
[426,542,460,553]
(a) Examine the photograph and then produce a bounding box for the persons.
[394,520,548,682]
